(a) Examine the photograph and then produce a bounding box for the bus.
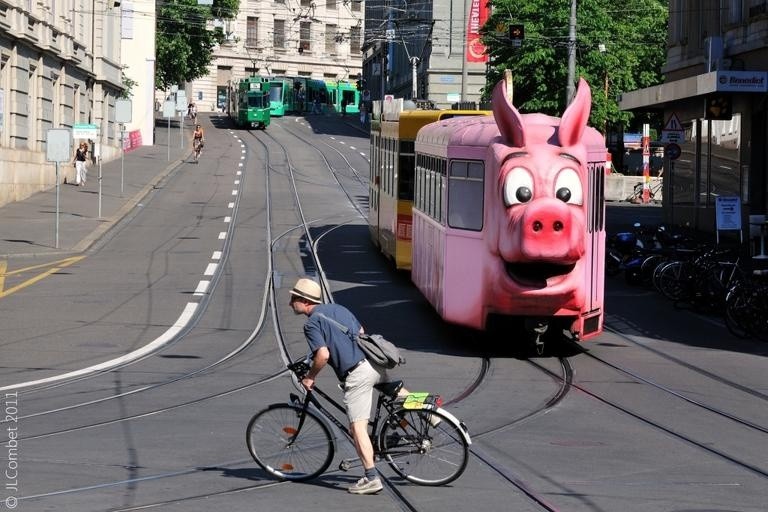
[229,74,363,131]
[366,106,495,274]
[409,75,615,347]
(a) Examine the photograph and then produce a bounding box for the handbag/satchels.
[354,333,399,368]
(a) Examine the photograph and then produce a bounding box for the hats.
[289,279,323,305]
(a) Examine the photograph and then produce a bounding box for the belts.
[345,360,365,374]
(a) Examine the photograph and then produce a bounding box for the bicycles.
[190,140,204,163]
[244,352,472,486]
[603,218,768,341]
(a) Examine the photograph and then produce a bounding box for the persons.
[286,277,444,495]
[192,125,205,156]
[340,97,348,113]
[309,96,321,116]
[358,102,369,130]
[186,102,199,125]
[72,142,89,187]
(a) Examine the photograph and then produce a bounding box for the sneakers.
[349,477,382,494]
[431,415,442,429]
